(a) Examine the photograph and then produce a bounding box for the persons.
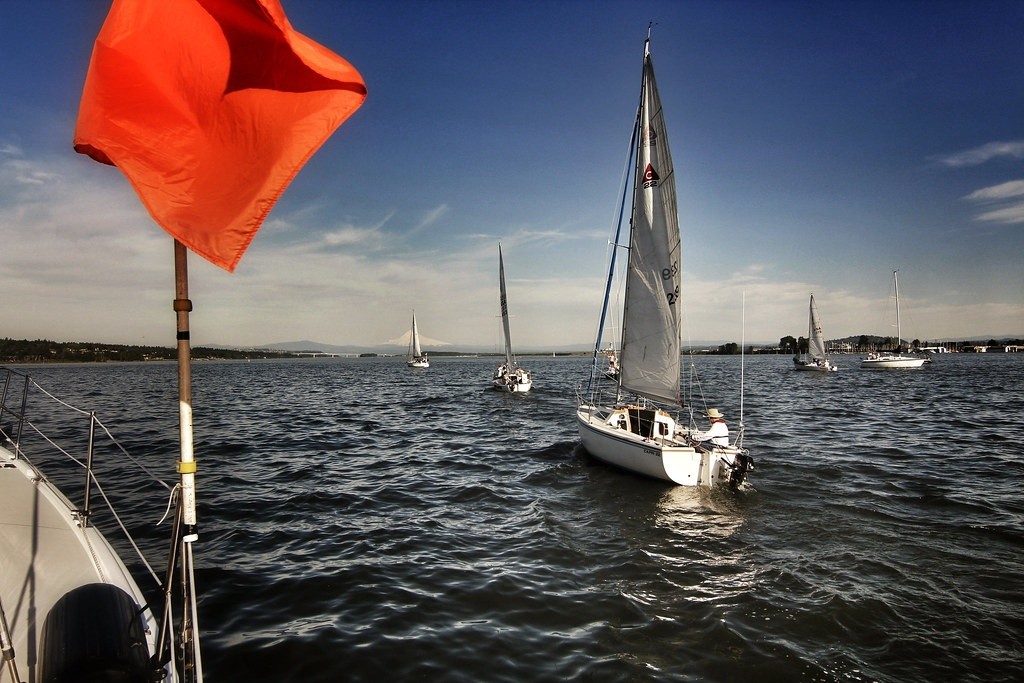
[606,353,620,375]
[688,408,729,451]
[496,364,525,390]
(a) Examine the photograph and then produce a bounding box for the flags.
[71,0,368,274]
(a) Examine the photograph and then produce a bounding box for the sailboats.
[577,23,758,494]
[790,292,839,373]
[492,242,533,393]
[406,308,431,368]
[858,268,924,369]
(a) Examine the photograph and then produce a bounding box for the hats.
[703,409,723,417]
